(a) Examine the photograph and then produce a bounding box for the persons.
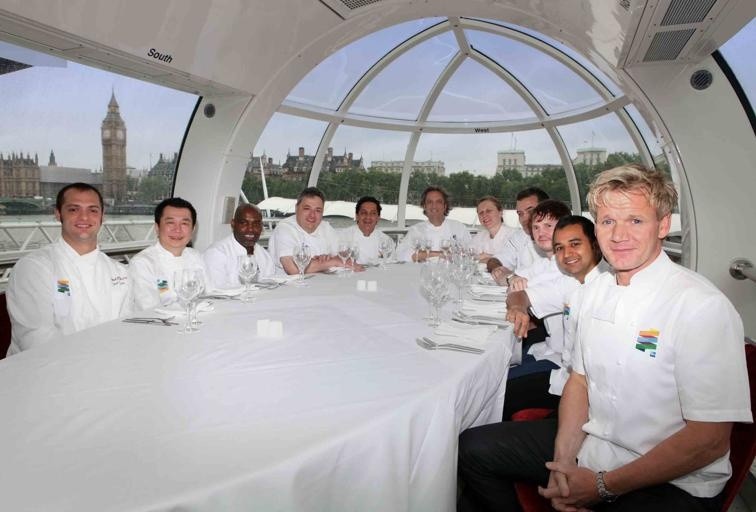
[386,187,476,260]
[5,182,144,358]
[477,186,552,349]
[268,187,368,277]
[504,200,573,382]
[201,203,277,291]
[334,198,396,264]
[126,198,213,317]
[502,215,604,424]
[454,161,753,510]
[468,196,521,265]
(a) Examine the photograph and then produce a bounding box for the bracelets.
[595,470,618,504]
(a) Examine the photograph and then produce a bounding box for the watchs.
[506,274,519,288]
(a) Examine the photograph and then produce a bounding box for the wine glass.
[412,238,480,329]
[172,267,206,336]
[336,239,360,278]
[378,238,394,272]
[235,254,261,304]
[293,244,314,287]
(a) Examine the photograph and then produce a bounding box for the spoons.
[255,280,288,285]
[415,336,485,355]
[121,315,179,326]
[199,294,241,300]
[452,310,511,324]
